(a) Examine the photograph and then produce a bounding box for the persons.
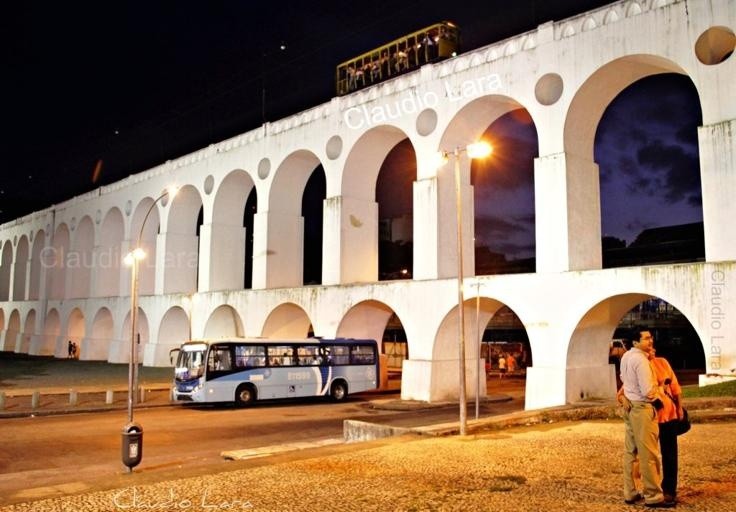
[616,348,684,501]
[214,350,331,366]
[67,341,75,359]
[72,343,77,360]
[498,354,506,380]
[619,324,678,508]
[507,353,520,377]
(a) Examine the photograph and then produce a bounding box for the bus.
[170,337,379,407]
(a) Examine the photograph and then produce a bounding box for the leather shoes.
[625,494,641,504]
[645,494,675,508]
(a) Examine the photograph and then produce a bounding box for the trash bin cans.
[121,422,144,467]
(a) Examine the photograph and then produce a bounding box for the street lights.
[422,141,492,435]
[123,185,179,422]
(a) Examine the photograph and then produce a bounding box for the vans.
[610,339,629,358]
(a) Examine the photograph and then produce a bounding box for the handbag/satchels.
[677,409,691,435]
